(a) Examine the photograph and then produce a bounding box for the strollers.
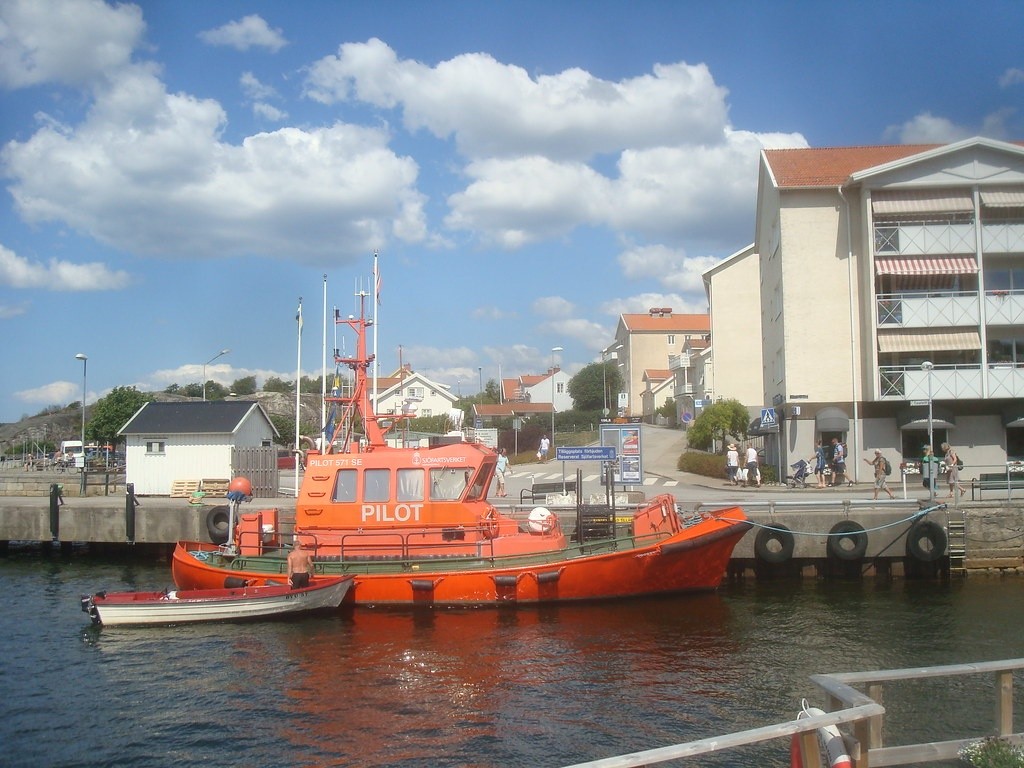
[787,458,813,488]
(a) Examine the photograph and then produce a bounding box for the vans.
[60,441,84,458]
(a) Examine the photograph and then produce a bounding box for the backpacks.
[879,457,891,475]
[949,450,964,470]
[837,443,848,458]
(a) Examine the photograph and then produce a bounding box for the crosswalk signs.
[761,409,775,424]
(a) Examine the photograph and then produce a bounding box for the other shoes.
[848,481,853,487]
[828,483,834,487]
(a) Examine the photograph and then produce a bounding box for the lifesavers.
[206,504,238,538]
[753,522,795,562]
[906,521,948,563]
[828,520,869,560]
[481,505,499,539]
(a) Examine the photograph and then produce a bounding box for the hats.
[727,444,736,450]
[922,445,927,448]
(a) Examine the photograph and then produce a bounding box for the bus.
[84,445,114,456]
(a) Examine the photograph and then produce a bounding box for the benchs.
[971,472,1024,501]
[520,481,577,511]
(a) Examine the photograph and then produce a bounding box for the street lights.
[550,346,564,447]
[920,360,935,501]
[603,344,624,418]
[75,354,89,497]
[202,348,231,401]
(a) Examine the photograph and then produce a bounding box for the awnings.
[1000,407,1024,427]
[897,406,956,431]
[748,416,779,436]
[815,406,850,433]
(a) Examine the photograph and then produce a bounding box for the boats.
[80,573,360,628]
[171,248,755,610]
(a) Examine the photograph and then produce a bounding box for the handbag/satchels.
[756,468,762,481]
[742,469,749,481]
[725,464,730,473]
[806,463,813,474]
[736,467,742,480]
[823,467,831,476]
[538,451,541,459]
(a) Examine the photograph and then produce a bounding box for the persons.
[536,435,551,463]
[807,439,826,489]
[915,444,939,499]
[48,450,75,468]
[495,448,514,498]
[863,449,896,500]
[287,541,315,590]
[25,453,33,471]
[939,443,966,499]
[827,438,854,487]
[742,443,761,487]
[727,444,740,486]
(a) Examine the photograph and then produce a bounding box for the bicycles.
[53,462,78,474]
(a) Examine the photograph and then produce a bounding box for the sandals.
[946,494,955,498]
[961,489,966,497]
